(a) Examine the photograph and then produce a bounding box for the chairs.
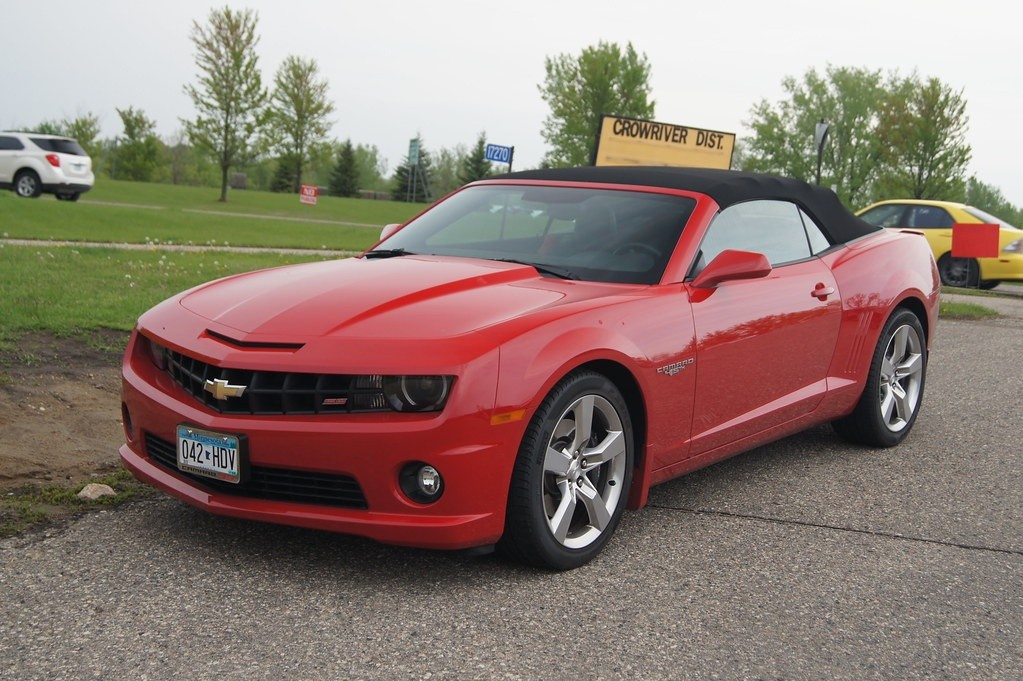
[641,205,688,248]
[543,204,617,261]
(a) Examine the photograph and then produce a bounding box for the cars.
[0,132,95,202]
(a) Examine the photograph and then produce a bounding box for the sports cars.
[848,198,1022,288]
[118,168,944,571]
